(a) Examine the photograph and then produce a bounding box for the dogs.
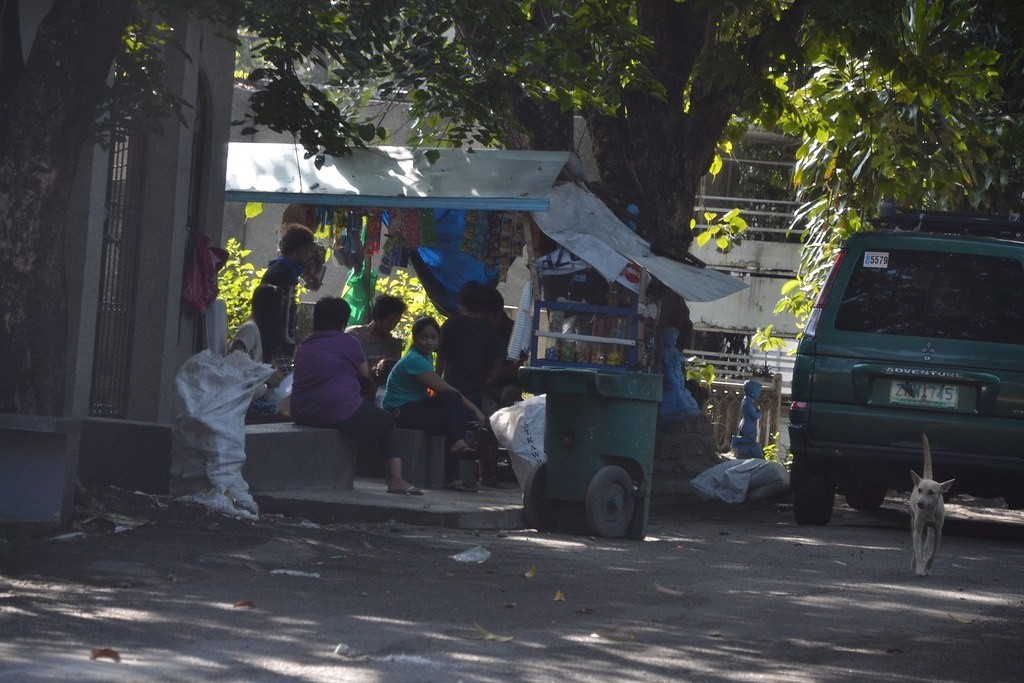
[909,433,956,577]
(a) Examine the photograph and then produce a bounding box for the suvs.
[788,204,1023,527]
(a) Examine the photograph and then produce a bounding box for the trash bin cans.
[513,362,667,542]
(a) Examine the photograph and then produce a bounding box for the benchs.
[79,415,353,493]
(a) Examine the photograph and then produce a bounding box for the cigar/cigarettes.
[482,427,487,430]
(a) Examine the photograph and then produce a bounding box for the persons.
[230,284,291,416]
[345,294,409,404]
[290,294,423,495]
[435,279,528,485]
[262,222,314,375]
[655,323,685,390]
[380,315,485,492]
[738,380,762,441]
